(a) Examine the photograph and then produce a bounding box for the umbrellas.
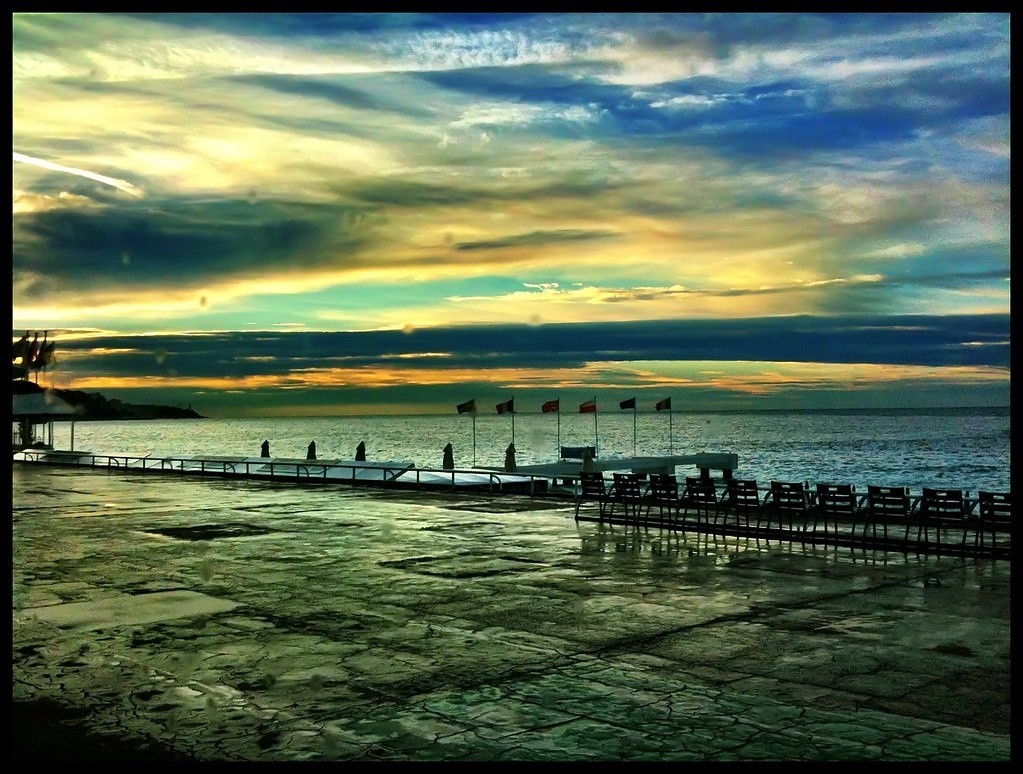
[443,441,454,469]
[260,440,270,457]
[505,442,516,473]
[355,441,366,462]
[307,440,316,460]
[582,446,593,472]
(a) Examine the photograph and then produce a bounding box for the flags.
[457,399,474,413]
[541,399,557,413]
[495,398,513,414]
[578,400,596,414]
[619,398,635,409]
[656,398,671,411]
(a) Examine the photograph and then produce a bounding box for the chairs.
[572,469,1013,567]
